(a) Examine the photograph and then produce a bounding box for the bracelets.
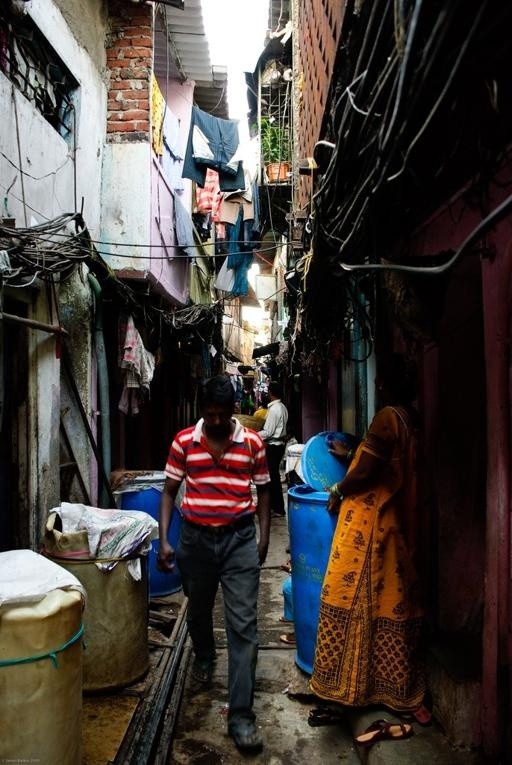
[345,448,355,462]
[329,483,343,498]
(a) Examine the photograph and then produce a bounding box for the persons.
[284,354,429,726]
[222,375,290,526]
[156,376,275,754]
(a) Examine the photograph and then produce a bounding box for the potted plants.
[252,118,293,184]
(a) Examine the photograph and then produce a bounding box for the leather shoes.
[308,705,344,727]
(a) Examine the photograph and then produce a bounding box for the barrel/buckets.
[285,442,308,487]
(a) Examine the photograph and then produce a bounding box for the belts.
[181,516,252,537]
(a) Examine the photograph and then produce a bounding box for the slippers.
[353,721,413,747]
[228,718,263,748]
[190,651,216,682]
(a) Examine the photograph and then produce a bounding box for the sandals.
[279,617,288,622]
[279,632,297,644]
